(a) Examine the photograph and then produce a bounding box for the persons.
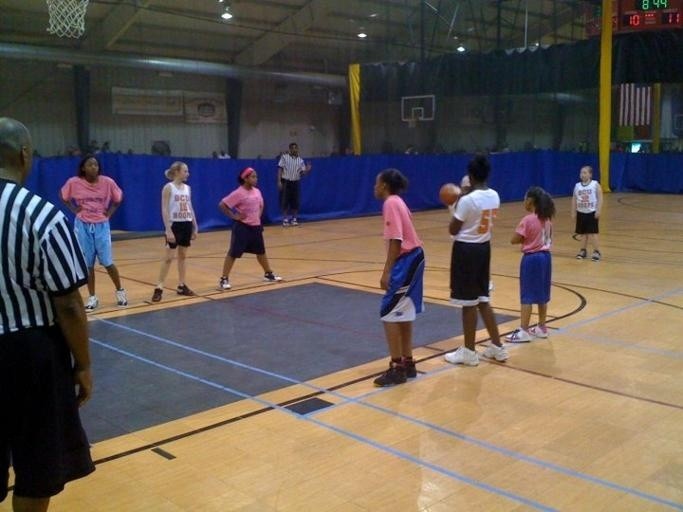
[570,167,602,262]
[33,139,683,159]
[0,118,94,512]
[277,143,310,226]
[215,166,282,290]
[444,158,508,367]
[505,188,556,343]
[152,162,197,301]
[58,154,128,310]
[373,169,425,385]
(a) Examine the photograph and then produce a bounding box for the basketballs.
[438,182,462,206]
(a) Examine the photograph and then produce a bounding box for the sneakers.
[482,344,509,361]
[402,360,417,378]
[504,329,531,342]
[591,249,600,262]
[373,362,407,386]
[444,346,479,367]
[529,326,547,338]
[576,249,586,259]
[283,219,288,226]
[291,218,298,226]
[85,270,284,309]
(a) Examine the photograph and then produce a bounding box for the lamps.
[453,36,466,53]
[354,21,368,38]
[219,0,233,22]
